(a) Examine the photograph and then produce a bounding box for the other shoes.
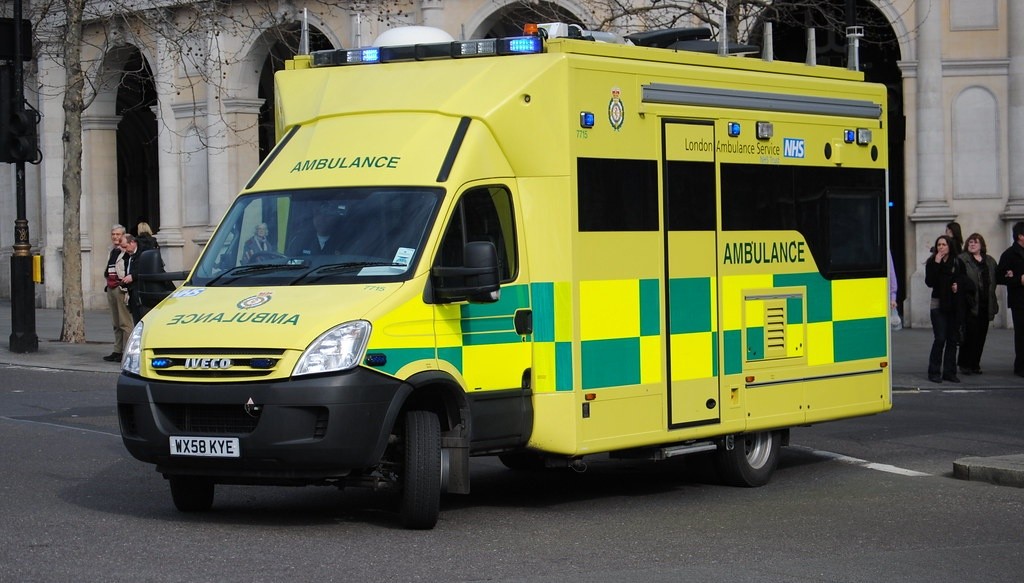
[928,373,942,383]
[959,366,982,376]
[942,375,961,383]
[103,352,123,362]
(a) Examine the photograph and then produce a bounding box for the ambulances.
[115,22,893,531]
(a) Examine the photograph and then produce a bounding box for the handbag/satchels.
[890,308,902,332]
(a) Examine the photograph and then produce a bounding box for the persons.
[295,207,356,257]
[889,251,898,311]
[244,222,276,265]
[925,221,1024,383]
[102,223,179,361]
